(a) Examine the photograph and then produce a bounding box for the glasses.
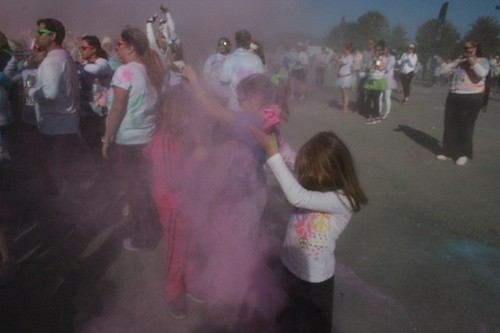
[35,29,52,36]
[117,40,127,48]
[462,47,474,51]
[79,45,95,51]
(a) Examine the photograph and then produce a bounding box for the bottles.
[25,75,35,107]
[91,77,101,97]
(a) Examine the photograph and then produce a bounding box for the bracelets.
[101,136,111,143]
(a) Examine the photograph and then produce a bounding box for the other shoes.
[456,156,468,166]
[0,256,23,286]
[187,290,208,303]
[436,153,455,160]
[122,238,154,251]
[364,117,381,125]
[168,297,188,319]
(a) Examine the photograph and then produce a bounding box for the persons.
[143,74,291,333]
[281,37,418,125]
[0,0,266,286]
[435,39,491,162]
[433,55,500,91]
[248,121,368,333]
